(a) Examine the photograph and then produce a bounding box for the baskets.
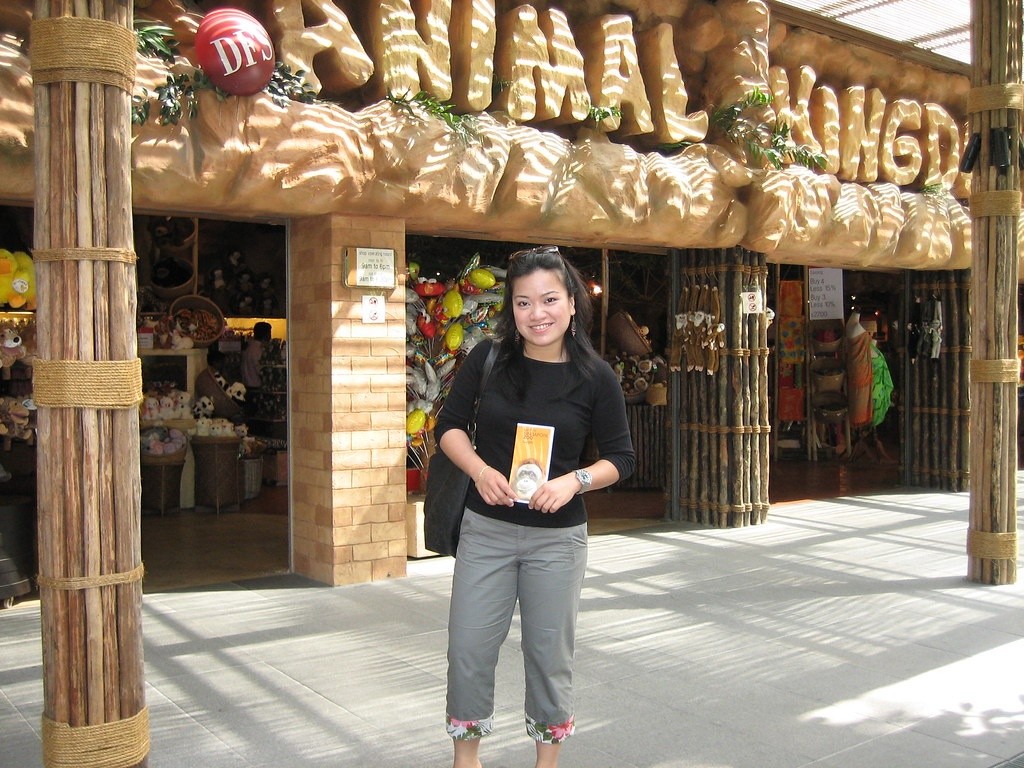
[150,257,196,301]
[810,360,845,392]
[811,391,847,422]
[164,218,196,252]
[141,426,188,460]
[170,295,224,347]
[195,366,241,418]
[610,362,655,405]
[607,309,653,359]
[809,318,845,354]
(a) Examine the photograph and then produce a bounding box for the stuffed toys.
[0,249,38,448]
[138,368,256,458]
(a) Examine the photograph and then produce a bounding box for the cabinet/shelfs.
[141,348,209,510]
[245,364,287,441]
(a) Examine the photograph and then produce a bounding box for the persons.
[434,245,636,768]
[242,321,272,398]
[846,313,894,427]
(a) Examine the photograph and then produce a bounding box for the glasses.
[509,245,573,288]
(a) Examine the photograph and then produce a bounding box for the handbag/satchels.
[423,339,502,559]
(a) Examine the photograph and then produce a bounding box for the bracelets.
[476,465,492,483]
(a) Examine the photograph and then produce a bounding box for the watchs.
[574,469,592,495]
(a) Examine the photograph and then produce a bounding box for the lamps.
[1009,138,1024,170]
[992,129,1011,167]
[959,135,981,174]
